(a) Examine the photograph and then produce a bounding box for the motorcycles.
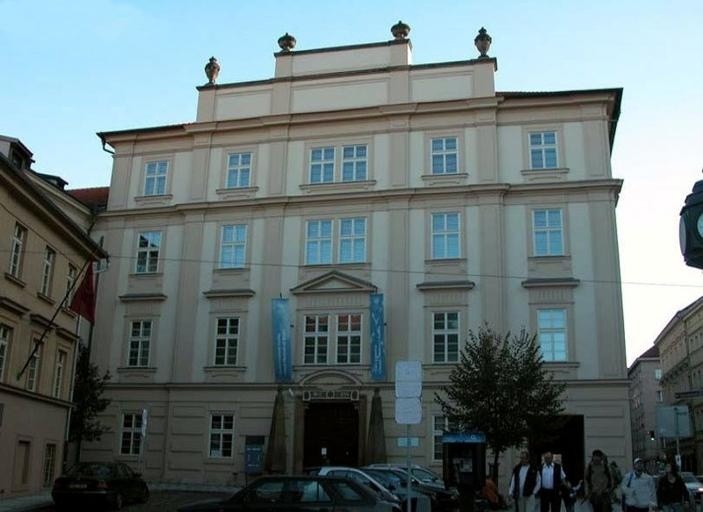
[476,494,508,510]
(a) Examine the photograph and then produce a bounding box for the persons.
[538,452,582,511]
[484,475,500,504]
[619,458,658,512]
[610,461,622,503]
[656,462,690,512]
[508,450,541,512]
[585,450,614,512]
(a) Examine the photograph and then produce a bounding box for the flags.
[69,261,95,327]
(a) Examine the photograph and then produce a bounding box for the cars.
[52,461,149,510]
[681,471,703,501]
[176,463,458,511]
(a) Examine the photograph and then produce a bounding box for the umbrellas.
[366,394,387,464]
[264,391,288,475]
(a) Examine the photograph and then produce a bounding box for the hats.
[633,457,643,465]
